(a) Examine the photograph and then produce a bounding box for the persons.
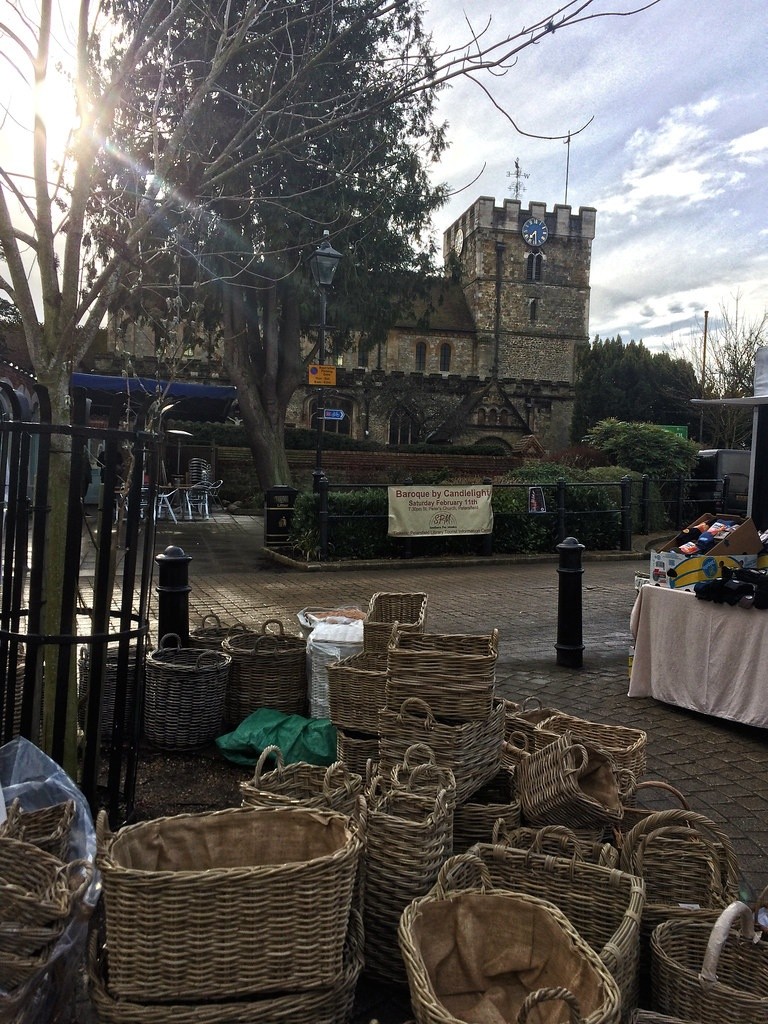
[82,451,124,518]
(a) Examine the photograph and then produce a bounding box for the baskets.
[0,592,768,1024]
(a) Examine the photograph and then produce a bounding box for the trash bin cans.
[263,484,299,548]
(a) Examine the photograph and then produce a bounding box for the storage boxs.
[657,512,764,556]
[649,549,758,592]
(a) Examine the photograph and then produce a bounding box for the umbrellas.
[70,373,235,429]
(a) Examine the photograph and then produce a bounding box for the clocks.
[521,218,549,247]
[454,228,464,256]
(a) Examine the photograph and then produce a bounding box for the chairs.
[114,458,224,519]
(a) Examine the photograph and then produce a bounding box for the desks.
[114,485,209,521]
[626,585,768,729]
